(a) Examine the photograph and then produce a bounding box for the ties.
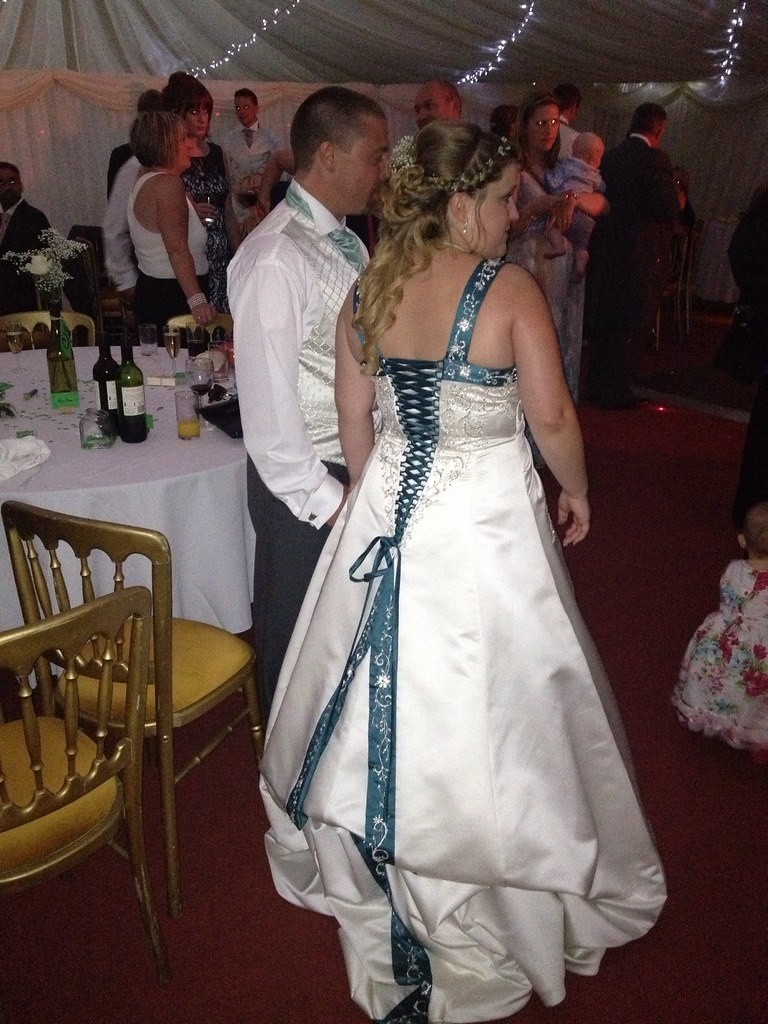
[285,186,366,276]
[242,128,255,149]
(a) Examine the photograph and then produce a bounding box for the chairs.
[1,493,270,917]
[0,585,170,994]
[652,220,705,348]
[0,311,96,352]
[167,314,233,352]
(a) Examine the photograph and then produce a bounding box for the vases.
[42,292,80,407]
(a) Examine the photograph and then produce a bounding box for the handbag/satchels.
[712,304,768,384]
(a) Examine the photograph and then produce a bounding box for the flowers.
[1,227,88,320]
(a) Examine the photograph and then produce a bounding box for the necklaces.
[441,243,465,252]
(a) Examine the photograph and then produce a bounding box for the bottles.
[93,330,120,439]
[115,335,147,443]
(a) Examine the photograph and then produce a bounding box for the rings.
[197,317,202,319]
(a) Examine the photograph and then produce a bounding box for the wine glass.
[164,325,182,378]
[186,358,215,409]
[5,321,27,374]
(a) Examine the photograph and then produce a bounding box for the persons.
[0,162,51,316]
[414,79,462,128]
[225,88,296,221]
[490,85,695,409]
[726,190,768,532]
[225,85,391,735]
[672,504,768,764]
[259,118,667,1024]
[105,73,230,350]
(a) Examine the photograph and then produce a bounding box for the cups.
[200,196,217,224]
[186,322,204,360]
[174,390,200,440]
[138,323,159,358]
[207,341,229,383]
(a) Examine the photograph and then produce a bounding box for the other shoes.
[610,395,649,408]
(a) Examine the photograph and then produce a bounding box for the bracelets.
[187,293,207,309]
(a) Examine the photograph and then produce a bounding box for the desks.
[685,212,752,305]
[0,344,257,695]
